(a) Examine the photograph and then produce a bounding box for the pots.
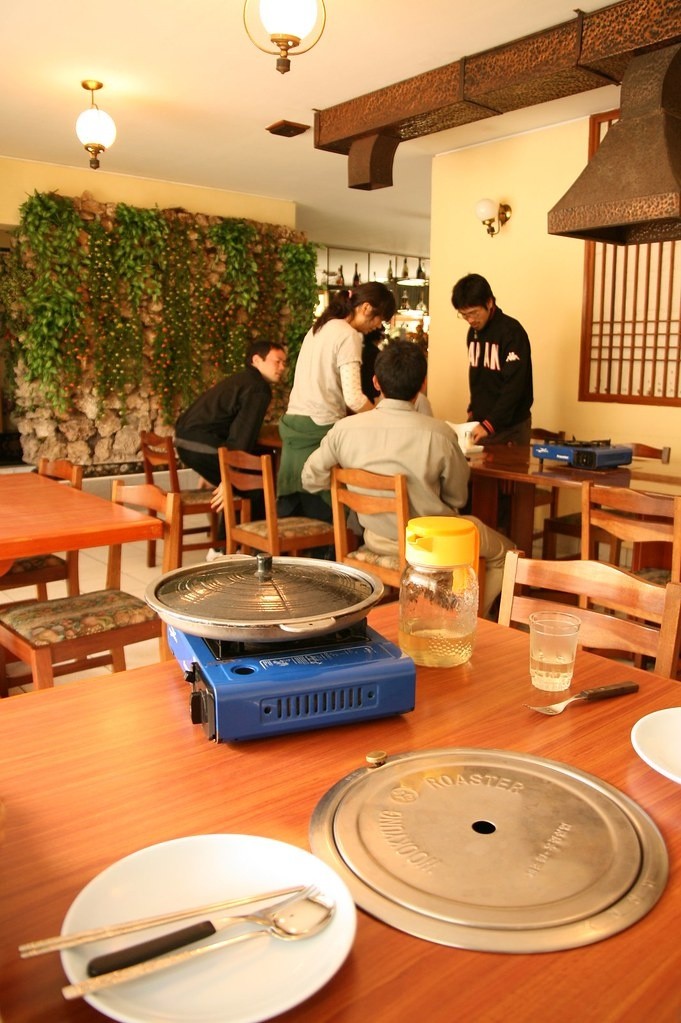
[145,554,384,643]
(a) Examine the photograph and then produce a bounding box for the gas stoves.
[166,621,416,744]
[533,434,632,471]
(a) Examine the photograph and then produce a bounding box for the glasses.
[457,301,483,319]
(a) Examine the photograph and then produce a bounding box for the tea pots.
[398,517,479,668]
[445,420,479,449]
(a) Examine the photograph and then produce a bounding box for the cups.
[529,611,582,691]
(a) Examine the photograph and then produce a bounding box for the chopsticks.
[19,885,306,959]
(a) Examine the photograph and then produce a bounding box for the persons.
[302,339,516,620]
[174,339,287,564]
[452,274,534,447]
[276,281,398,559]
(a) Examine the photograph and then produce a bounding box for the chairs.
[538,438,673,610]
[532,425,567,554]
[480,550,681,680]
[218,445,359,558]
[0,457,89,601]
[578,479,681,671]
[331,464,484,617]
[0,475,189,691]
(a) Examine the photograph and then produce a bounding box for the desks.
[0,467,167,566]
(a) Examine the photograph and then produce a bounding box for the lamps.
[243,0,328,74]
[75,75,119,173]
[473,196,512,239]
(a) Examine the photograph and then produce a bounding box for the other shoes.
[206,547,223,561]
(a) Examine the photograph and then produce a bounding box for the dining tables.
[256,416,544,475]
[466,452,681,557]
[0,599,681,1023]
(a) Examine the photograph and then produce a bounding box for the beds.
[138,427,254,570]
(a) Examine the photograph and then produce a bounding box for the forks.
[522,681,639,714]
[88,885,321,976]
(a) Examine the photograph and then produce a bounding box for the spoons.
[62,897,335,1000]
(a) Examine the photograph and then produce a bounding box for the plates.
[631,707,681,783]
[457,446,483,452]
[59,834,357,1023]
